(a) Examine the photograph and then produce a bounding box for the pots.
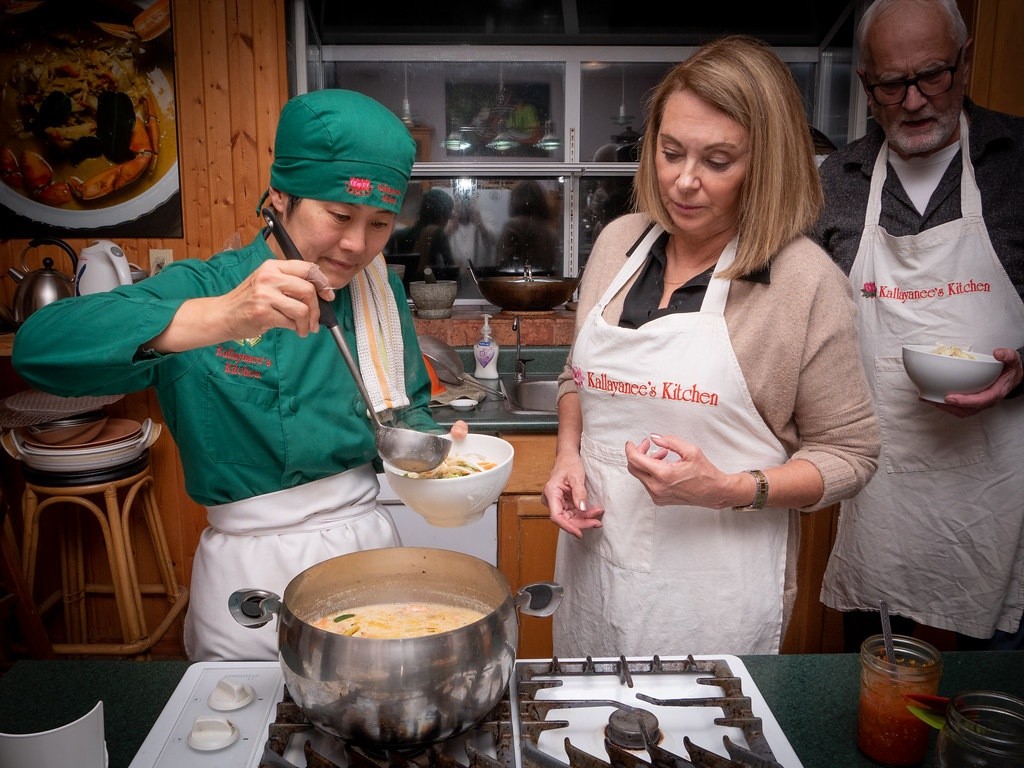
[228,545,564,749]
[466,257,585,311]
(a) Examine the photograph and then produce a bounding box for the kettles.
[8,238,79,334]
[73,240,133,298]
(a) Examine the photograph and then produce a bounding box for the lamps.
[397,62,423,129]
[609,63,637,125]
[438,62,562,156]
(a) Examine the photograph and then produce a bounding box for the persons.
[805,0,1024,653]
[11,87,468,665]
[542,35,881,659]
[387,143,643,299]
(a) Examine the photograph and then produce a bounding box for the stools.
[16,463,189,662]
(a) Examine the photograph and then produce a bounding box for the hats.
[421,187,454,219]
[255,88,417,217]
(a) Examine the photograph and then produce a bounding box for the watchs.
[732,469,769,515]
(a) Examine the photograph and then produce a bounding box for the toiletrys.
[472,313,500,379]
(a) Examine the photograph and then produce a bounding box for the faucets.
[511,315,535,381]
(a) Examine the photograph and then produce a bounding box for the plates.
[21,418,144,472]
[0,48,181,231]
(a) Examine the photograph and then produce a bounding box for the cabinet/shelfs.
[397,124,435,228]
[495,429,834,659]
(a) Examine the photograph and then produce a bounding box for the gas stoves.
[126,652,805,768]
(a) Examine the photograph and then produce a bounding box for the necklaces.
[663,279,694,288]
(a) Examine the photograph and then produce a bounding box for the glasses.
[862,43,968,107]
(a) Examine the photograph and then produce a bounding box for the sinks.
[512,381,559,411]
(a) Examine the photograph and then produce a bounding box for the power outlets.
[149,248,174,270]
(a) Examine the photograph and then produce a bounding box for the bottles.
[857,634,944,768]
[937,689,1024,768]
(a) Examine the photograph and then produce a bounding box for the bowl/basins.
[448,398,478,413]
[26,414,107,447]
[381,433,515,529]
[901,344,1004,405]
